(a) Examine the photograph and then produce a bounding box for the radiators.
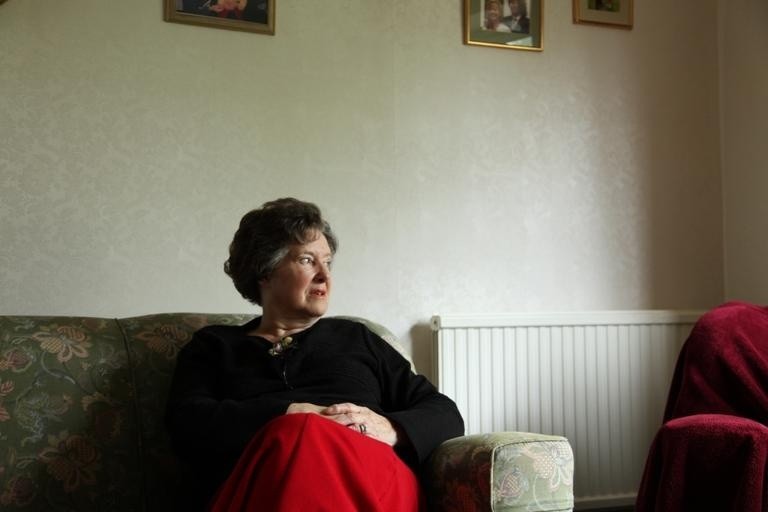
[429,311,704,512]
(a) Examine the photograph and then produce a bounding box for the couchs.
[0,313,573,512]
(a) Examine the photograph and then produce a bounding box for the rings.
[358,423,366,434]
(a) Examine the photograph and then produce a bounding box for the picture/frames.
[462,0,545,52]
[571,0,634,31]
[163,0,275,35]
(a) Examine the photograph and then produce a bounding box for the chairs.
[635,301,768,512]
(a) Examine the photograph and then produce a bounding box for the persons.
[200,1,250,21]
[501,0,531,34]
[156,194,470,510]
[481,0,510,32]
